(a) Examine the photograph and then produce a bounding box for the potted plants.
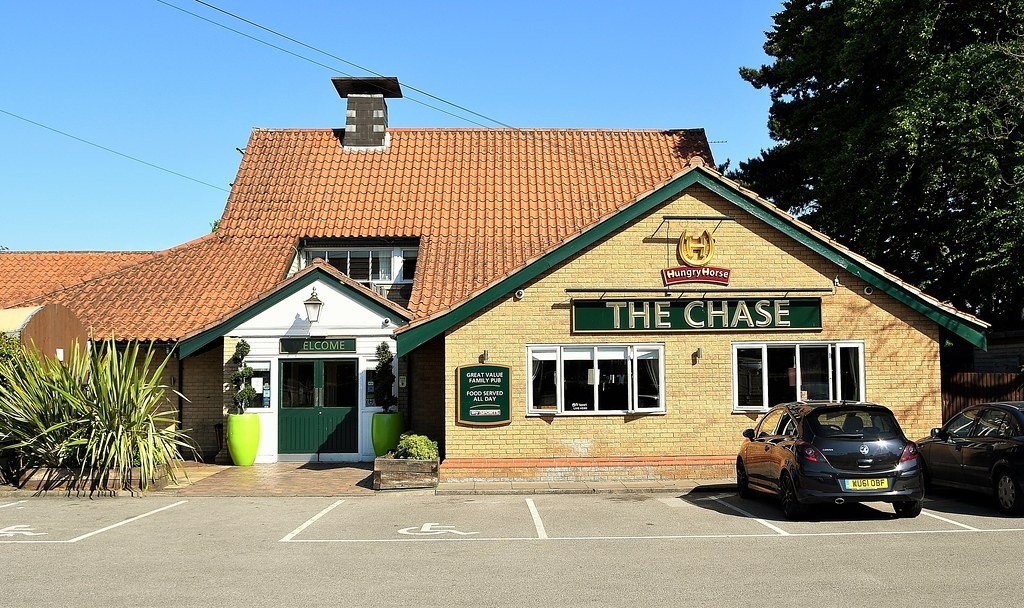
[0,328,206,495]
[373,431,440,490]
[227,340,259,466]
[371,341,403,456]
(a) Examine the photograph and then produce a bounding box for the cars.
[734,398,925,521]
[911,401,1024,515]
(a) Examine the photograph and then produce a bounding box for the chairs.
[843,416,864,434]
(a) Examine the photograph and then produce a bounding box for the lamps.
[484,349,488,359]
[304,287,322,321]
[695,348,702,358]
[171,376,175,385]
